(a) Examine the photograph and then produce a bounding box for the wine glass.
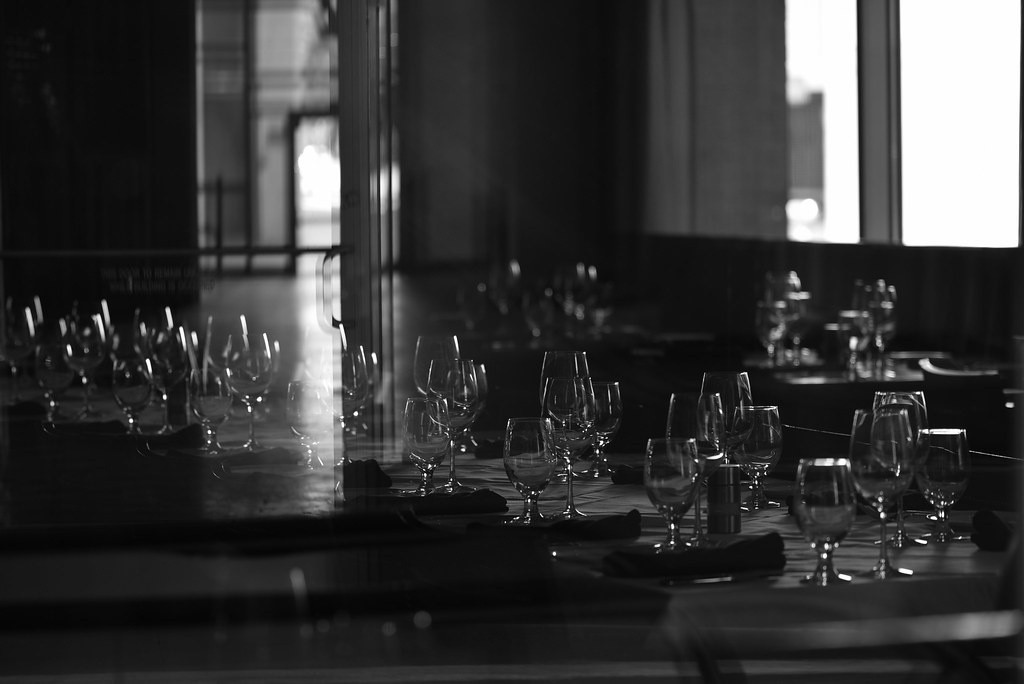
[540,377,596,484]
[730,406,781,513]
[666,392,726,536]
[826,274,900,383]
[644,438,701,552]
[872,390,928,547]
[850,408,916,579]
[541,350,595,484]
[327,332,490,497]
[792,458,856,586]
[0,294,324,479]
[503,417,556,526]
[592,381,622,475]
[752,264,824,377]
[917,428,972,542]
[700,372,754,467]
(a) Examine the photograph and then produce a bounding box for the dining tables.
[1,321,1024,684]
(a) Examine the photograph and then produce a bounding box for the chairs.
[918,358,1014,457]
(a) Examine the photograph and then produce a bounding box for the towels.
[351,488,508,514]
[2,401,205,450]
[601,528,787,580]
[492,506,642,536]
[339,456,395,487]
